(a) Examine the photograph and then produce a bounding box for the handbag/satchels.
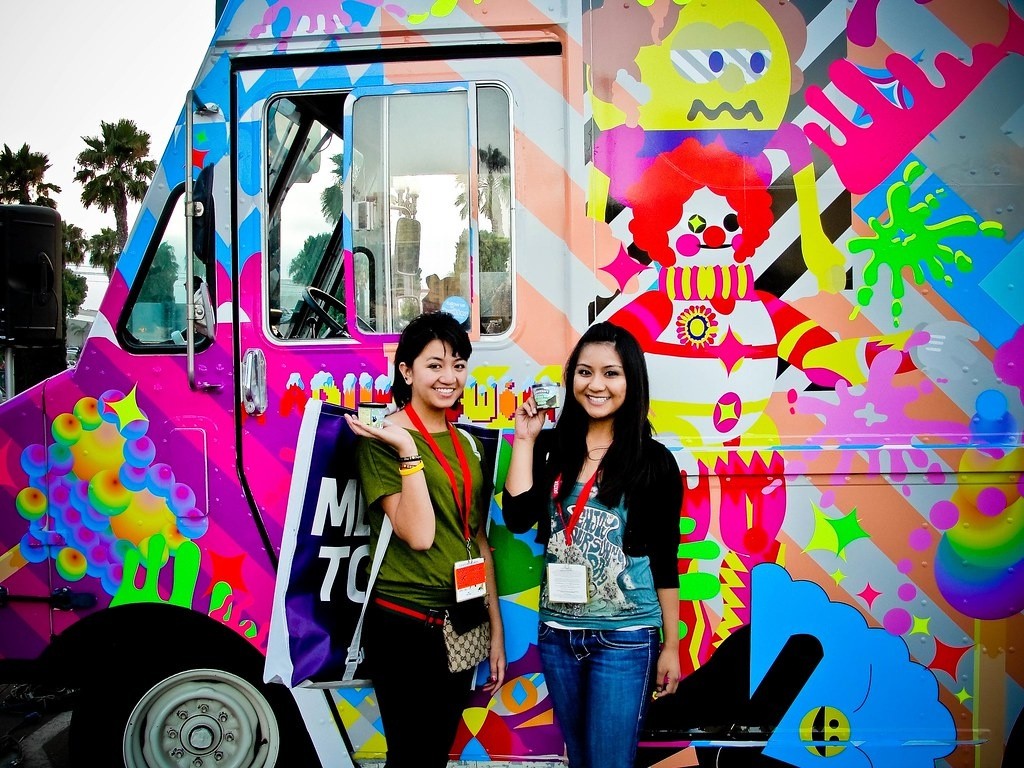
[443,596,491,674]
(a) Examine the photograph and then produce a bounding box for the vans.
[0,203,67,404]
[0,0,1024,767]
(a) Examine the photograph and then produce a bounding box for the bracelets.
[398,455,424,476]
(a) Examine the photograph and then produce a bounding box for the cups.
[357,403,388,430]
[533,382,561,409]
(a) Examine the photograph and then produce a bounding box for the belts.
[375,598,444,626]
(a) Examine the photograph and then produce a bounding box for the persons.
[501,321,683,768]
[344,312,506,768]
[159,276,204,344]
